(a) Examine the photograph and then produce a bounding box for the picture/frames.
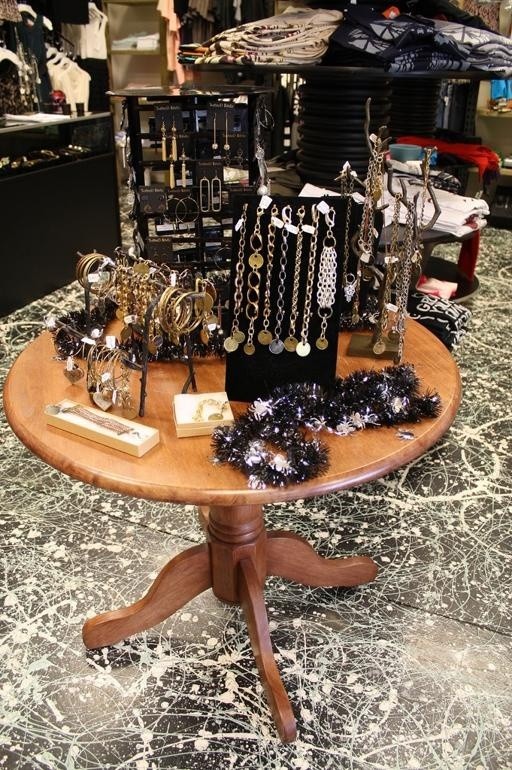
[376,223,485,302]
[3,302,461,746]
[183,61,512,198]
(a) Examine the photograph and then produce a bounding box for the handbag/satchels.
[53,325,84,359]
[159,286,205,346]
[86,343,134,410]
[75,253,116,295]
[221,204,336,357]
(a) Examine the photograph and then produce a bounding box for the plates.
[102,0,173,188]
[472,106,512,219]
[0,111,120,314]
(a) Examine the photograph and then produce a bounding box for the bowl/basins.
[373,192,415,364]
[340,141,383,304]
[412,149,432,267]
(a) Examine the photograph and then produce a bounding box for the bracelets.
[388,144,422,162]
